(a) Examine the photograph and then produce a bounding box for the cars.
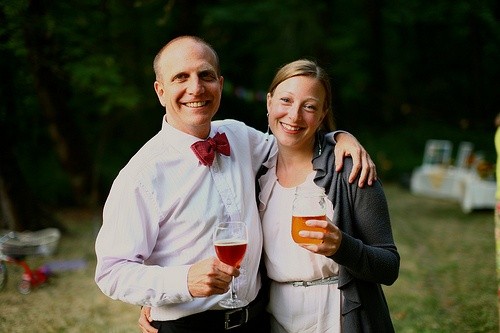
[410,141,497,216]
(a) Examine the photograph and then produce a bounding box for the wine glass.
[214,221,249,308]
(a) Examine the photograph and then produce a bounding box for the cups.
[291,186,327,245]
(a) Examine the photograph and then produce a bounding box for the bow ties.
[190,131,231,166]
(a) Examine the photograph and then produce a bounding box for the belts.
[279,276,338,287]
[160,294,268,330]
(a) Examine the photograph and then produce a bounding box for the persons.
[94,35,377,333]
[139,60,400,333]
